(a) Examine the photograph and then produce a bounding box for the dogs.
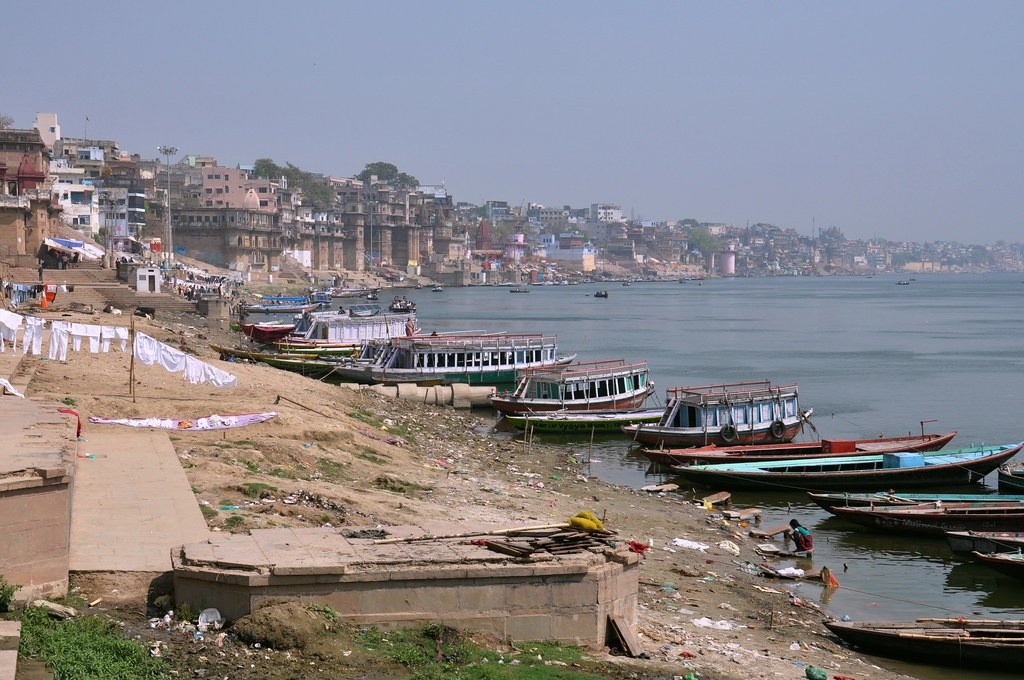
[112,309,122,316]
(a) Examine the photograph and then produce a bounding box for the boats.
[640,418,959,473]
[997,467,1024,496]
[505,406,666,432]
[389,303,417,313]
[943,528,1024,557]
[620,378,813,450]
[669,441,1024,495]
[490,358,656,417]
[807,490,1024,537]
[208,287,577,388]
[594,292,608,298]
[432,287,444,292]
[822,616,1024,676]
[970,549,1024,584]
[509,290,530,293]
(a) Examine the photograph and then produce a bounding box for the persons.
[405,317,414,337]
[116,257,134,268]
[787,519,813,552]
[338,306,345,314]
[351,344,359,359]
[49,248,79,265]
[218,284,222,295]
[38,267,43,280]
[310,286,313,291]
[431,331,438,336]
[166,273,195,300]
[393,295,416,309]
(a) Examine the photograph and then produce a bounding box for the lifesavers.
[770,420,786,439]
[720,425,737,442]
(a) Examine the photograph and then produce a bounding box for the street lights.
[157,145,179,270]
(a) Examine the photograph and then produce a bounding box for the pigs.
[136,306,155,315]
[134,310,151,321]
